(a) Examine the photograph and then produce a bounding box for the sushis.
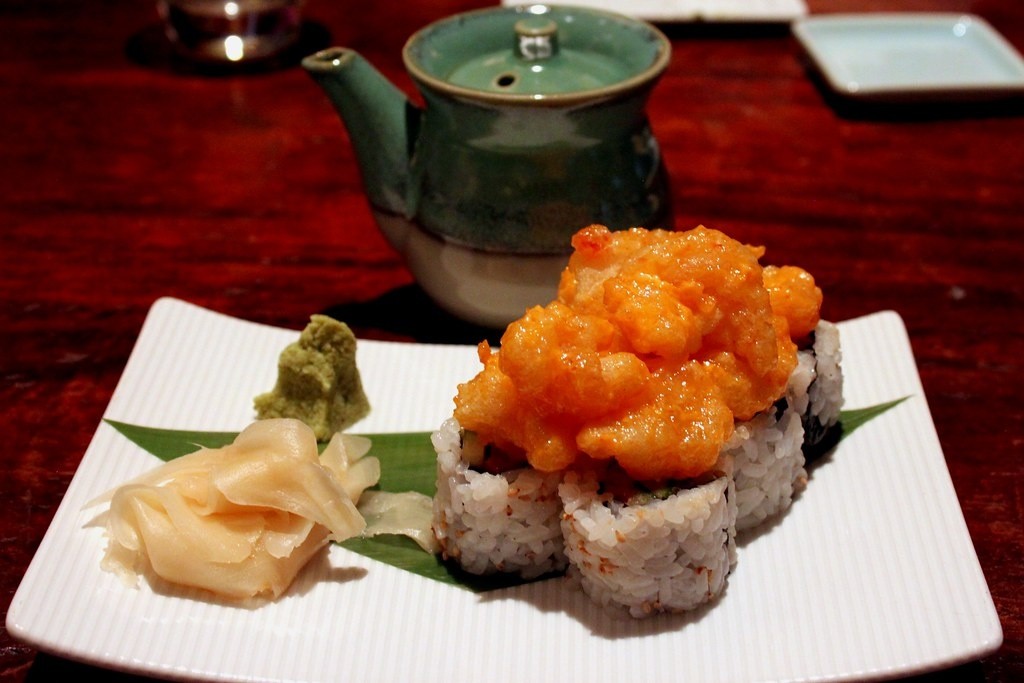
[429,319,844,618]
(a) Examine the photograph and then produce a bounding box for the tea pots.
[304,4,675,330]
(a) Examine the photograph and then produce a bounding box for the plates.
[502,0,809,22]
[794,11,1024,94]
[9,298,1006,683]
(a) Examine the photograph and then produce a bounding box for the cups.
[157,0,298,69]
